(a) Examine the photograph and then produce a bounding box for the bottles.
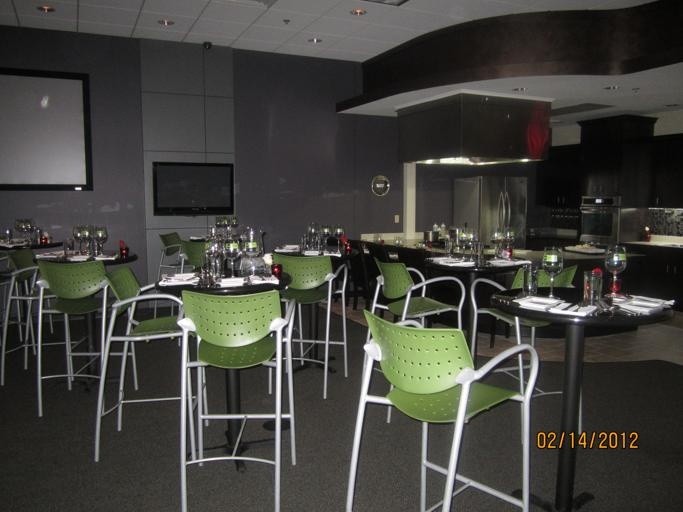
[432,216,446,241]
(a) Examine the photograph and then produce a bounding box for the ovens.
[579,195,622,244]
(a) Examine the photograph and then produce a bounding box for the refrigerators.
[451,176,528,249]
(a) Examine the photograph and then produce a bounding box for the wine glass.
[14,217,34,243]
[306,224,345,254]
[72,223,108,257]
[604,246,627,297]
[448,225,473,262]
[542,246,564,301]
[490,228,516,261]
[200,216,265,290]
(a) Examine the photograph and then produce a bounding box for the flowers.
[584,268,602,302]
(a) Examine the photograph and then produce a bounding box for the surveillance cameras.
[204,42,212,49]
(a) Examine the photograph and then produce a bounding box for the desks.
[489,288,673,512]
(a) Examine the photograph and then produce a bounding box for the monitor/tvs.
[152,161,235,216]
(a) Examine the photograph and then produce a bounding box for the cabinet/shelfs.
[619,243,682,312]
[534,114,682,209]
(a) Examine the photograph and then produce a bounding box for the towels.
[611,294,675,318]
[511,294,597,317]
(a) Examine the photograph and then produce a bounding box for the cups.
[445,241,457,263]
[522,263,538,298]
[63,239,74,255]
